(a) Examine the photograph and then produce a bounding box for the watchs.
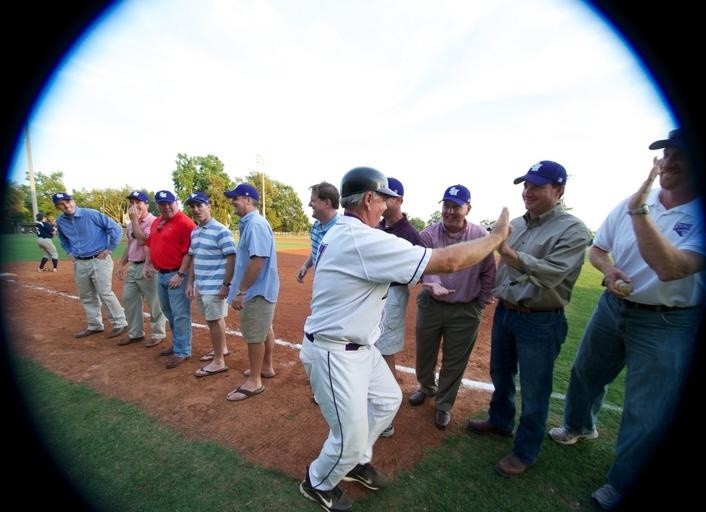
[222,281,231,285]
[626,205,650,215]
[236,290,250,295]
[176,269,185,278]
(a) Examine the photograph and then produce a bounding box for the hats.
[439,184,471,206]
[53,193,72,205]
[341,166,401,198]
[186,192,210,204]
[155,190,177,204]
[125,191,149,204]
[649,129,695,150]
[514,160,567,187]
[224,184,259,201]
[387,178,404,197]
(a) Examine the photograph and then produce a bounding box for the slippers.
[194,364,229,377]
[226,384,265,401]
[243,367,276,379]
[200,349,230,362]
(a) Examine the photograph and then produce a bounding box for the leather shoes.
[468,416,514,437]
[494,453,529,477]
[166,356,191,368]
[75,329,104,338]
[108,326,127,338]
[145,338,166,347]
[117,335,145,346]
[409,390,427,406]
[435,407,454,430]
[160,345,174,356]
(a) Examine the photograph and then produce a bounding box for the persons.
[144,190,197,367]
[297,165,510,512]
[36,213,59,273]
[549,128,704,507]
[53,192,128,338]
[223,183,280,402]
[369,177,425,438]
[115,191,167,349]
[186,193,235,378]
[298,181,344,282]
[470,161,592,477]
[407,184,496,429]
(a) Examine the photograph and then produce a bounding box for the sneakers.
[590,484,624,511]
[299,464,353,512]
[548,425,599,445]
[37,267,48,273]
[343,463,387,490]
[52,268,61,273]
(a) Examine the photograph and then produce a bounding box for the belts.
[498,298,564,314]
[73,252,101,260]
[133,260,145,264]
[621,298,701,311]
[158,267,179,273]
